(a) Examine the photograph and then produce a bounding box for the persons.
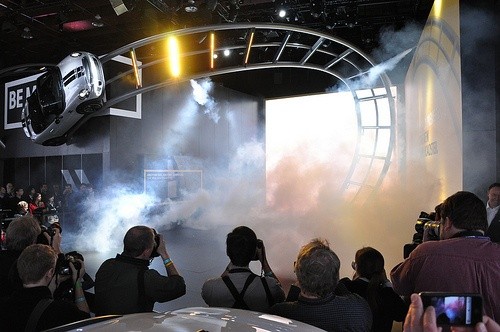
[390,190,499,324]
[484,183,500,241]
[201,226,286,309]
[339,246,408,331]
[0,184,124,228]
[268,238,373,332]
[93,226,186,314]
[48,250,95,299]
[438,312,451,324]
[401,291,500,331]
[1,244,91,332]
[1,216,65,289]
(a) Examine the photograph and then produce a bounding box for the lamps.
[243,27,255,67]
[129,47,143,89]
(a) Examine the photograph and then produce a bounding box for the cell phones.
[419,292,483,327]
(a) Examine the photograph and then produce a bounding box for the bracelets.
[261,271,275,276]
[162,257,174,267]
[75,282,83,289]
[75,296,86,303]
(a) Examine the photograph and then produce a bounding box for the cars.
[21,51,106,146]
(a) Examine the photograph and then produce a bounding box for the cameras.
[417,220,440,238]
[55,253,81,276]
[41,223,62,245]
[151,228,160,258]
[285,284,301,302]
[252,239,262,261]
